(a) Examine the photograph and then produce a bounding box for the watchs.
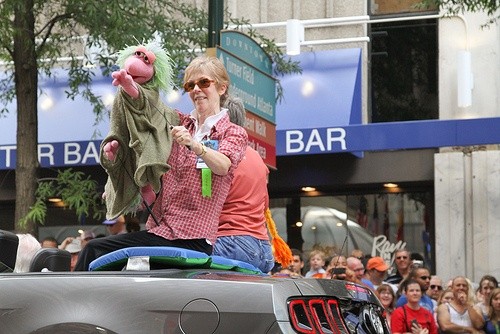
[196,142,209,157]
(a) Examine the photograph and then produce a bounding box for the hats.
[366,257,388,272]
[59,237,81,253]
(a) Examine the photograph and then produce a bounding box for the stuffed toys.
[101,46,179,234]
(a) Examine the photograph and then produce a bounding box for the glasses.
[397,257,407,259]
[184,78,214,92]
[421,276,431,280]
[431,285,442,290]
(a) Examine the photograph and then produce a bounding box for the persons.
[72,55,249,273]
[212,97,274,276]
[394,267,434,321]
[486,288,500,334]
[276,244,500,334]
[437,276,484,334]
[375,284,395,331]
[391,279,438,334]
[41,211,126,273]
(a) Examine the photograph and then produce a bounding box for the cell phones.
[331,268,346,274]
[413,260,423,268]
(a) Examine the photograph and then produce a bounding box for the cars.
[0,230,390,334]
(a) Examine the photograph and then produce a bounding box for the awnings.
[0,50,500,168]
